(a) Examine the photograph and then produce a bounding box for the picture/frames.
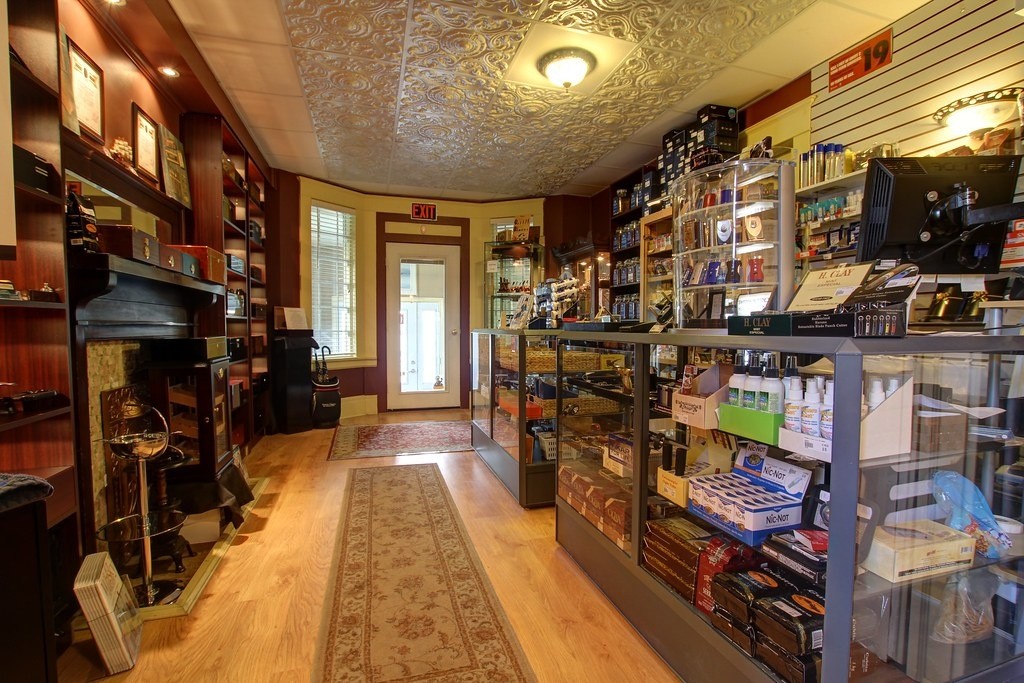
[131,101,161,182]
[67,34,106,146]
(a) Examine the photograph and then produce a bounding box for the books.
[71,552,141,675]
[771,530,829,562]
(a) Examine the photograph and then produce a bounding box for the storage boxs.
[182,253,201,280]
[914,412,966,453]
[862,516,975,583]
[97,223,159,268]
[658,104,739,198]
[167,244,227,286]
[152,335,228,359]
[158,243,183,273]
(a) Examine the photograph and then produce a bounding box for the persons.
[515,296,528,319]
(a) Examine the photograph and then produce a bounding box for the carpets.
[326,420,478,461]
[310,463,539,683]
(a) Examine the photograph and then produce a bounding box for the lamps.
[536,47,598,88]
[931,88,1024,141]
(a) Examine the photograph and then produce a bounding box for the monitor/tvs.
[854,156,1020,275]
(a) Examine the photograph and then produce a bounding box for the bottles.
[535,277,581,328]
[613,183,643,320]
[729,351,899,442]
[799,142,856,189]
[800,189,864,227]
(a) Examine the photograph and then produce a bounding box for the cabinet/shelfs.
[609,164,657,321]
[183,114,268,458]
[672,160,797,320]
[483,241,544,329]
[0,0,82,683]
[469,328,631,509]
[796,166,866,262]
[554,329,1024,683]
[147,360,234,480]
[640,206,673,322]
